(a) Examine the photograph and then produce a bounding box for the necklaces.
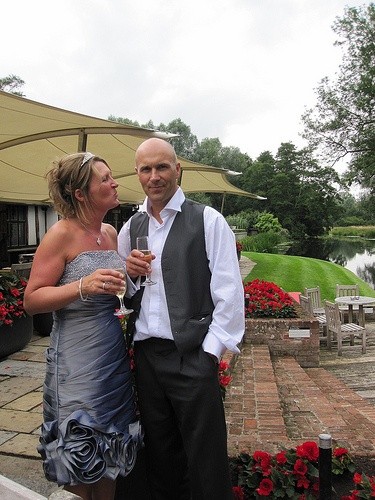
[96,235,102,245]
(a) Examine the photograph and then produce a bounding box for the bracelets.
[79,277,88,301]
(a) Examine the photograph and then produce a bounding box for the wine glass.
[109,259,134,316]
[136,236,158,287]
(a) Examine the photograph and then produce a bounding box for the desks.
[335,296,375,340]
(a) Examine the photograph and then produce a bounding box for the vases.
[0,315,34,359]
[33,312,54,336]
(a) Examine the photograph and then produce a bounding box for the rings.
[103,282,105,290]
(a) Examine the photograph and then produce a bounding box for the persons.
[116,137,244,500]
[23,151,146,500]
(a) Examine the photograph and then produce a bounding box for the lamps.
[318,433,332,450]
[246,294,250,298]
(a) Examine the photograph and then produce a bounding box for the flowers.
[0,268,29,328]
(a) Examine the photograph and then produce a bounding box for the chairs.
[298,283,367,357]
